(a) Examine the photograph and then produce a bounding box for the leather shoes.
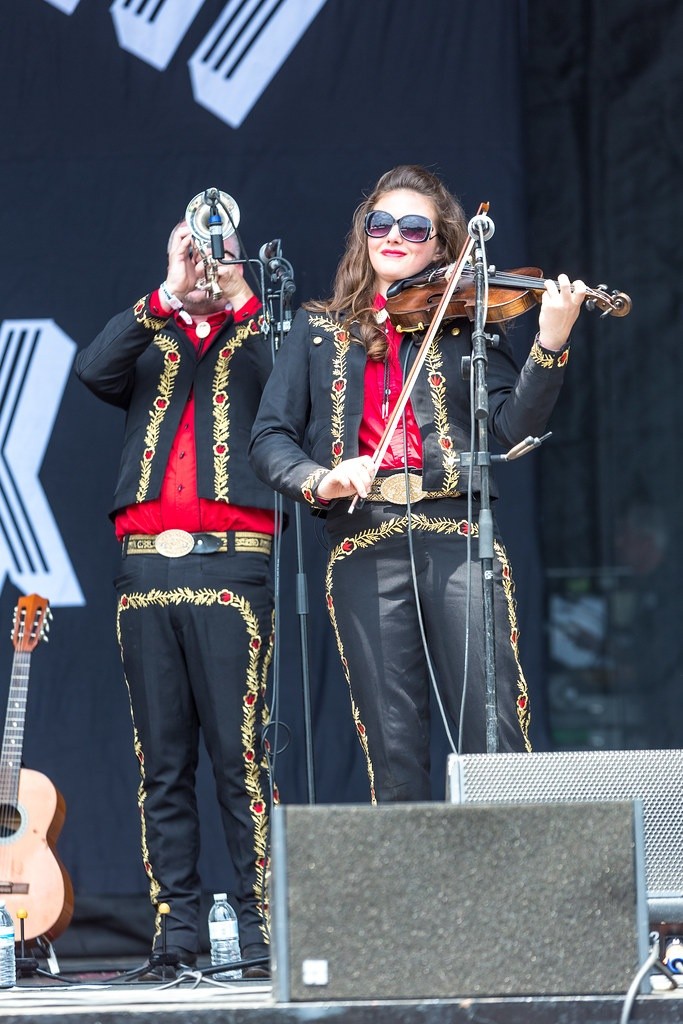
[138,957,199,981]
[241,961,271,978]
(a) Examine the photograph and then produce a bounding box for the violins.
[384,259,633,333]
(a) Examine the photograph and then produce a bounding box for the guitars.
[0,591,77,949]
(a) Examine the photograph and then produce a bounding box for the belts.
[347,473,460,505]
[121,528,273,558]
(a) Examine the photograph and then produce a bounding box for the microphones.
[202,187,220,206]
[258,238,283,264]
[468,216,495,242]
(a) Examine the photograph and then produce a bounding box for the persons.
[250,164,588,806]
[74,220,287,979]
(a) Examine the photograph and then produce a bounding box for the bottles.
[0,900,16,989]
[208,893,243,980]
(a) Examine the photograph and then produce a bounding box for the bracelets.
[159,281,193,325]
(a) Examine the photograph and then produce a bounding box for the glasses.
[364,210,432,244]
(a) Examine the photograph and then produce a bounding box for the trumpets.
[184,189,242,302]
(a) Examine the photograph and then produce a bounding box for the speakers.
[267,749,683,1005]
